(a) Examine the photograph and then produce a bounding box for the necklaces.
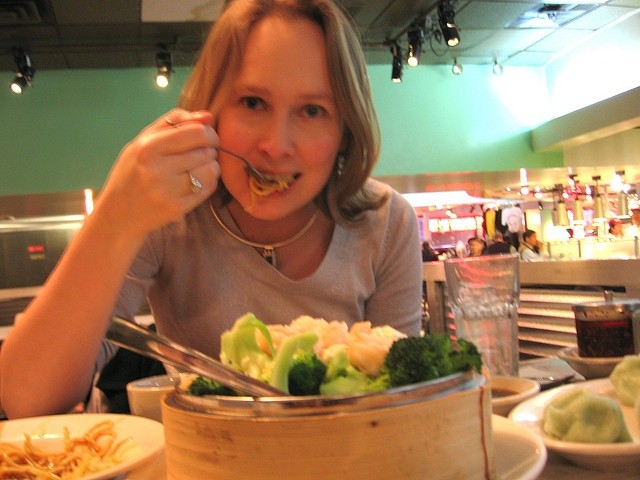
[206,196,323,271]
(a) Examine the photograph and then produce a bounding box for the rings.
[185,169,202,195]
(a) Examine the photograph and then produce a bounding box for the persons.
[422,230,546,262]
[607,204,640,238]
[0,0,422,418]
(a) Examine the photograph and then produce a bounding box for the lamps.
[11,51,33,94]
[390,42,403,83]
[574,196,584,224]
[550,207,560,222]
[155,48,171,88]
[619,189,630,216]
[593,193,606,224]
[405,23,420,67]
[437,4,460,47]
[451,61,463,76]
[492,61,502,76]
[555,201,570,225]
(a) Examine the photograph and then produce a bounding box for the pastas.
[1,421,134,480]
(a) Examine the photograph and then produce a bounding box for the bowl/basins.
[123,370,197,422]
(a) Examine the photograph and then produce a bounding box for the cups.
[443,252,522,379]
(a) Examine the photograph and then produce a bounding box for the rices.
[255,316,407,374]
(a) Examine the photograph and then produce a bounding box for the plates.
[491,413,550,480]
[1,408,170,479]
[557,343,640,374]
[485,375,542,416]
[505,374,640,470]
[516,357,579,387]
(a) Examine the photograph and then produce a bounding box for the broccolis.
[290,355,328,397]
[385,331,451,387]
[323,351,386,395]
[453,337,481,373]
[274,333,318,394]
[219,312,274,387]
[189,376,233,398]
